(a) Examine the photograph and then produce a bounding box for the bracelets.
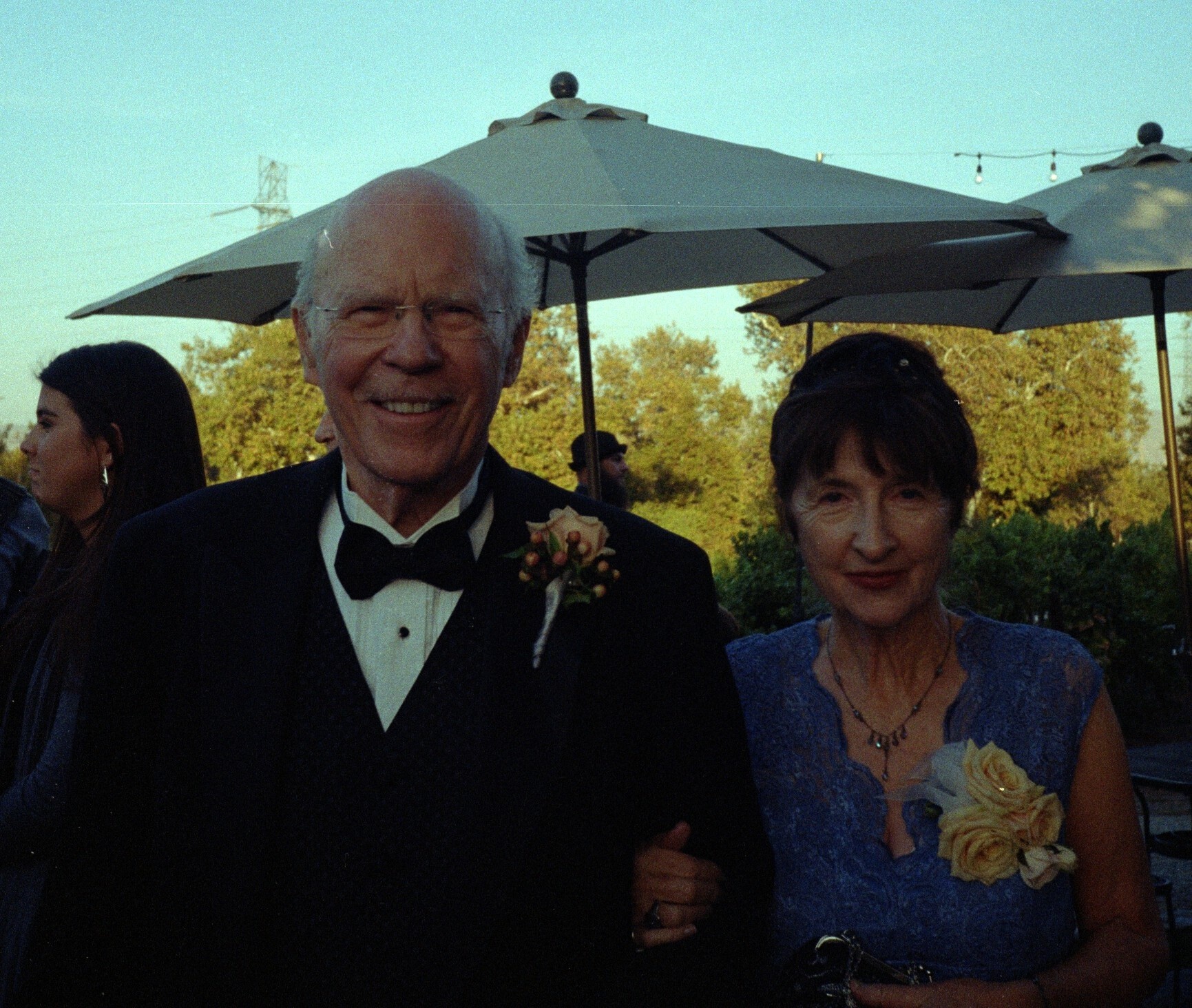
[1032,976,1054,1008]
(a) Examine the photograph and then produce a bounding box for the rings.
[644,903,662,929]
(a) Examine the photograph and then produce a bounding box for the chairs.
[1125,772,1192,1008]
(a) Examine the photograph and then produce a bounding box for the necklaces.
[824,609,951,780]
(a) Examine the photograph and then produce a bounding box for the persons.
[1,171,1164,1008]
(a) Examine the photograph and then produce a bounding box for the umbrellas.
[64,72,1192,570]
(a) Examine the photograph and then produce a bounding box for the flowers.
[911,736,1081,895]
[503,498,621,669]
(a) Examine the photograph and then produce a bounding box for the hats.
[568,431,627,470]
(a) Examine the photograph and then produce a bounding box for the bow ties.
[333,460,488,599]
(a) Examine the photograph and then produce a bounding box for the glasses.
[803,936,916,1002]
